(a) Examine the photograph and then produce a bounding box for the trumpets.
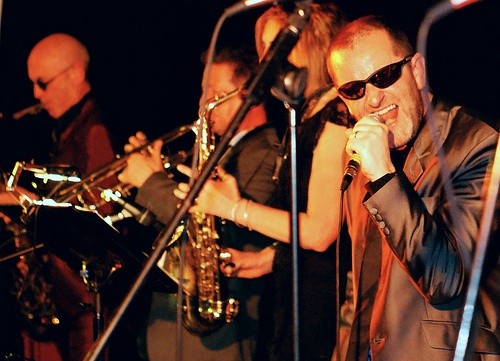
[53,118,198,210]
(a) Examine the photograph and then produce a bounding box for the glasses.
[28,66,73,91]
[336,54,413,100]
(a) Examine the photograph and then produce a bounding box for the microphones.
[340,151,362,190]
[109,194,154,227]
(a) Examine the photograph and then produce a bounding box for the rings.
[354,131,359,138]
[194,196,198,204]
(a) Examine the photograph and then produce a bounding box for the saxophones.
[0,101,71,344]
[151,82,244,337]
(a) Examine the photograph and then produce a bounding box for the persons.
[173,0,358,361]
[116,42,287,361]
[325,18,500,361]
[0,32,122,361]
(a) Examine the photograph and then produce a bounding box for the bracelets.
[235,197,253,232]
